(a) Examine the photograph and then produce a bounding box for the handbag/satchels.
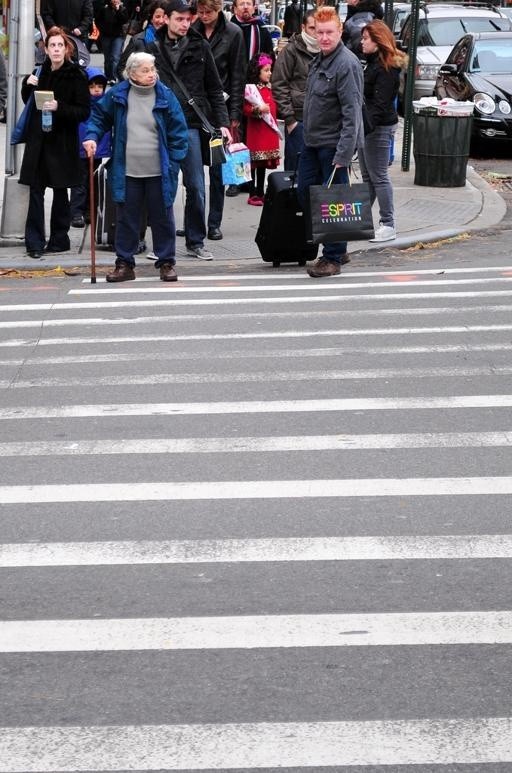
[11,92,34,145]
[198,120,227,167]
[309,179,375,245]
[222,145,253,185]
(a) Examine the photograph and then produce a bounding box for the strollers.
[61,26,91,68]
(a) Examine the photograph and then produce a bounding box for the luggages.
[93,126,147,253]
[255,151,319,268]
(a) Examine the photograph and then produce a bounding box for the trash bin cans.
[412,111,474,187]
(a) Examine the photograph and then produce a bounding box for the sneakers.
[225,184,241,195]
[70,215,86,227]
[307,251,350,277]
[106,260,135,282]
[159,263,177,281]
[248,195,263,204]
[184,245,214,260]
[368,222,396,242]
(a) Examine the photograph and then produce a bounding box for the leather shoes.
[208,227,223,240]
[29,250,44,258]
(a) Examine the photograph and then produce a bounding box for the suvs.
[395,1,512,118]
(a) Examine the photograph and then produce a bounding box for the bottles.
[41,101,53,132]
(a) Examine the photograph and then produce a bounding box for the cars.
[431,31,512,154]
[258,0,512,64]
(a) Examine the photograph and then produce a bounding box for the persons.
[1,1,409,282]
